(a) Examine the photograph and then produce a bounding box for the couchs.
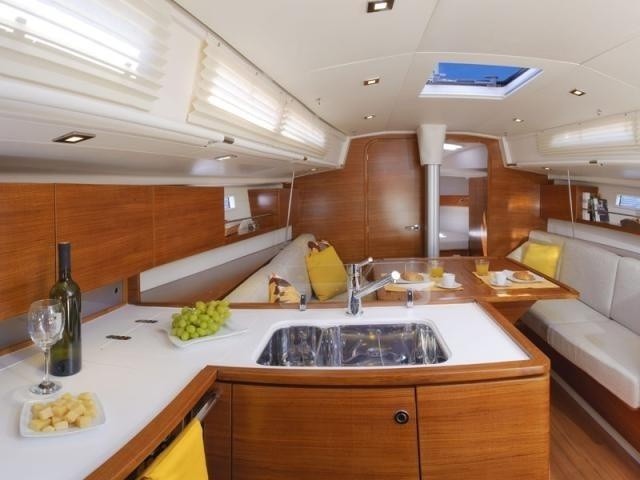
[214,233,377,304]
[504,230,640,462]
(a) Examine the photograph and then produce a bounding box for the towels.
[136,418,211,480]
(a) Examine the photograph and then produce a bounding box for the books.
[581,191,609,223]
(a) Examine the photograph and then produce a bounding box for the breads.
[513,270,533,280]
[401,272,424,281]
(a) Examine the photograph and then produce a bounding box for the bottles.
[47,241,83,377]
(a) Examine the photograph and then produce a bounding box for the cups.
[474,259,490,276]
[427,259,444,279]
[442,272,456,287]
[494,271,507,284]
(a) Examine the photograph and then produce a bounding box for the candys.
[26,392,96,432]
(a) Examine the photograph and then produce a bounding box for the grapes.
[169,300,231,339]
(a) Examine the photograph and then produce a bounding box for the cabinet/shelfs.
[231,384,420,480]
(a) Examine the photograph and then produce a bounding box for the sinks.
[255,326,320,367]
[317,321,451,366]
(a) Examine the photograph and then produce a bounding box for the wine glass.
[26,297,67,396]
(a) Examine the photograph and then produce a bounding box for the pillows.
[268,238,350,305]
[522,242,561,279]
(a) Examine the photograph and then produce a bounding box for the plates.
[491,280,512,287]
[392,271,428,284]
[436,282,463,289]
[18,391,107,439]
[506,271,546,283]
[156,315,250,350]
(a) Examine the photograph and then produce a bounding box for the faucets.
[346,255,401,313]
